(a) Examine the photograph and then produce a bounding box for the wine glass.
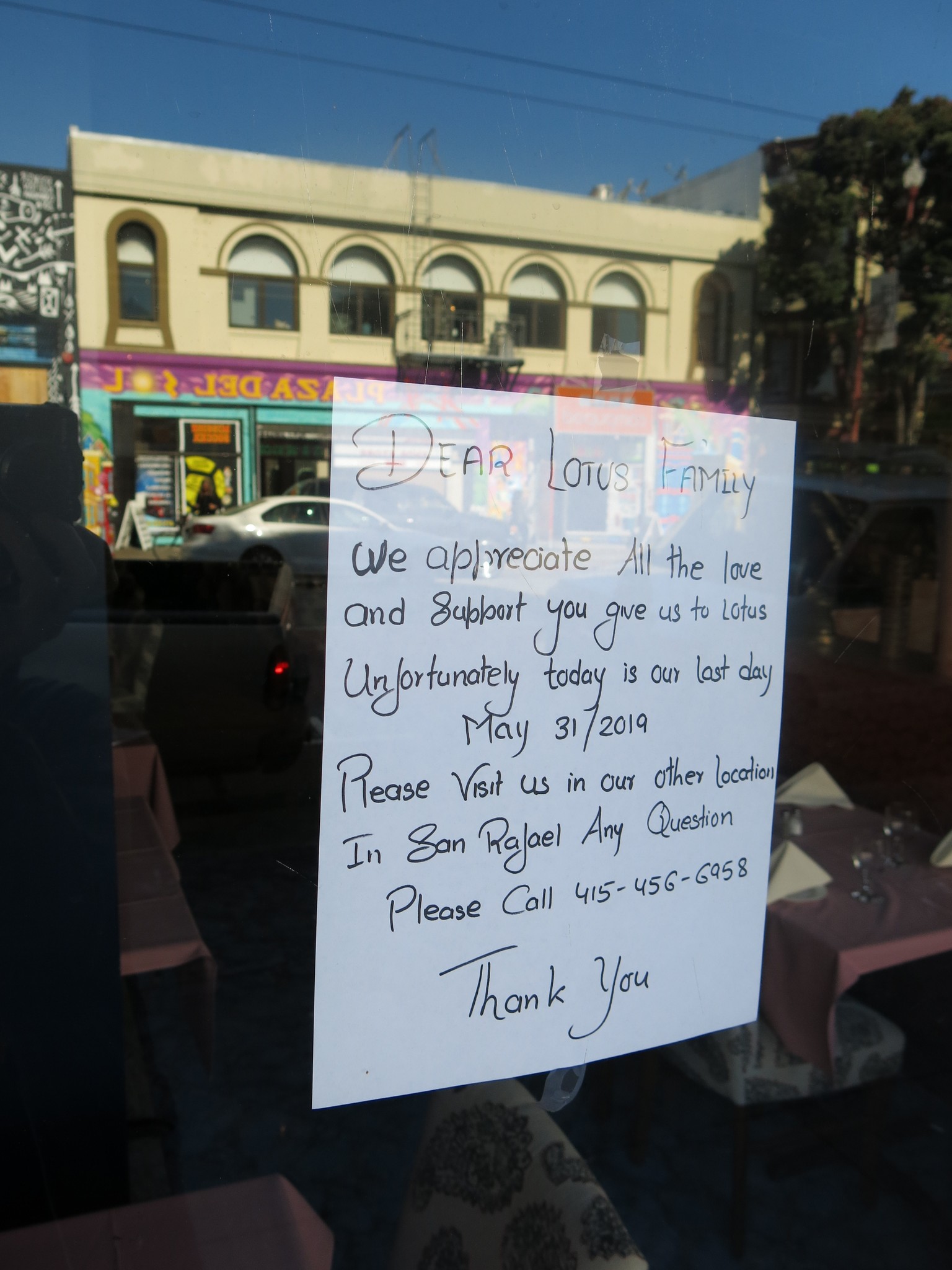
[849,838,879,901]
[880,806,908,867]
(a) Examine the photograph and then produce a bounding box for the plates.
[782,885,829,904]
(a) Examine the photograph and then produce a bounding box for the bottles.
[780,803,802,837]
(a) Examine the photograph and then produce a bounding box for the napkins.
[929,832,951,869]
[773,761,853,811]
[764,839,833,905]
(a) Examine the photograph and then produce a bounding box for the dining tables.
[0,1171,337,1270]
[106,710,181,847]
[111,795,219,999]
[751,804,952,1075]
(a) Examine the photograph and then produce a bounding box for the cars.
[284,475,530,564]
[180,494,481,574]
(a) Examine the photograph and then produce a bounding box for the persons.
[194,478,222,516]
